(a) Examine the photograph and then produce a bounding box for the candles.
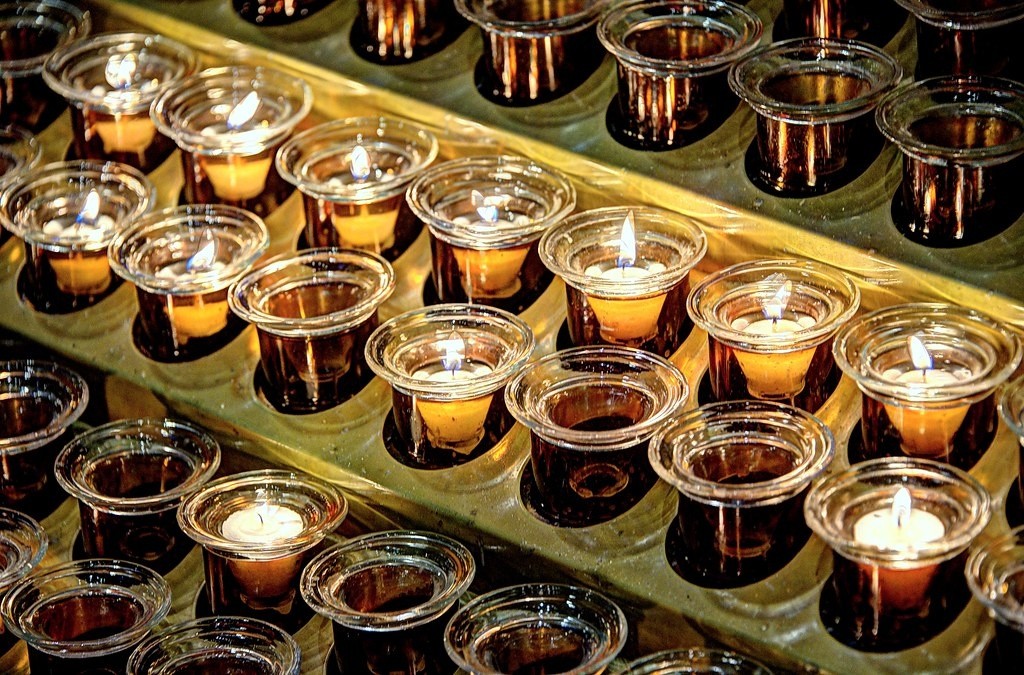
[365,303,534,468]
[177,469,348,636]
[109,204,266,356]
[43,30,199,174]
[538,205,706,358]
[406,156,576,311]
[686,260,861,415]
[149,65,314,219]
[833,302,1023,468]
[0,159,155,315]
[803,456,993,641]
[276,117,438,259]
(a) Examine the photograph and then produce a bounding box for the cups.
[3,358,770,673]
[1,2,1024,568]
